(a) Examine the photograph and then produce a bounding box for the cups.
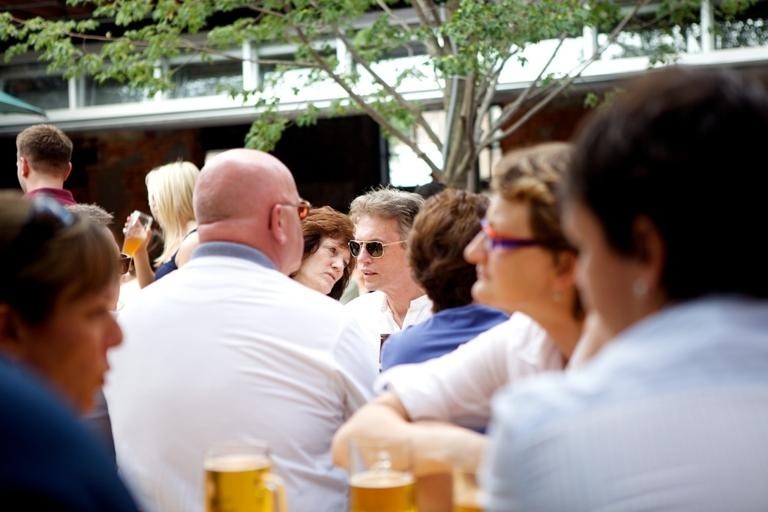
[201,441,283,512]
[117,210,152,259]
[345,437,418,512]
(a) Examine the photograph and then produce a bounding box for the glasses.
[288,200,310,220]
[478,220,567,252]
[120,254,131,273]
[0,190,76,286]
[347,238,407,259]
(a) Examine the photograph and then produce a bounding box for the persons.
[1,63,768,512]
